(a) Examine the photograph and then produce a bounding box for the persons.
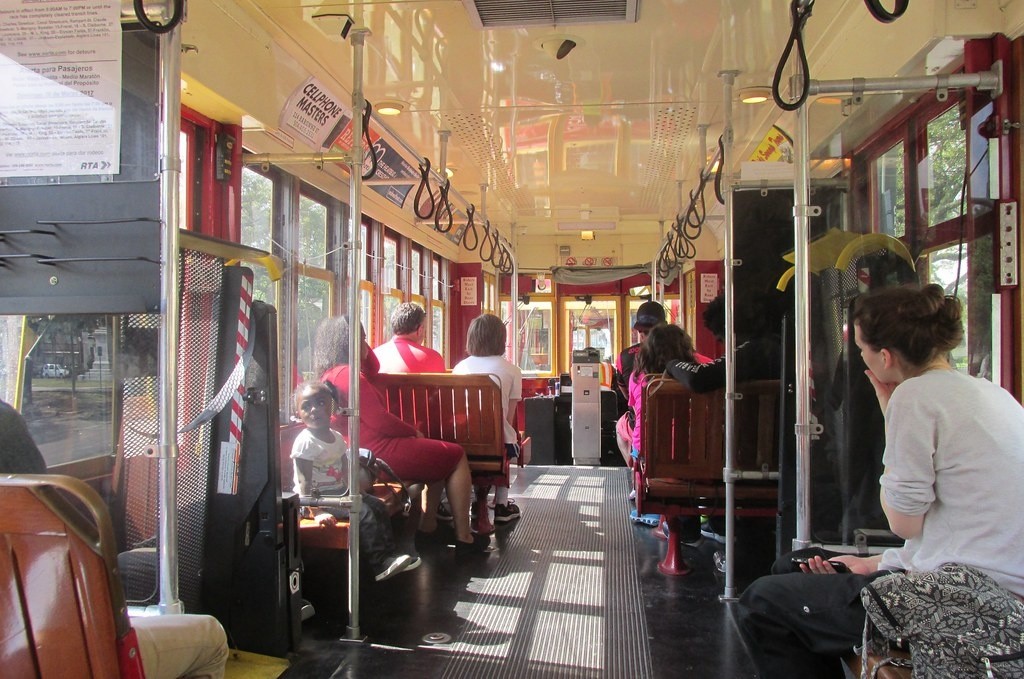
[315,315,491,560]
[721,284,1024,679]
[372,302,453,520]
[615,302,666,498]
[290,380,421,581]
[0,400,229,679]
[662,296,769,547]
[451,313,522,521]
[628,324,697,526]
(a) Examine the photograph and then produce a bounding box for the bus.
[508,297,680,376]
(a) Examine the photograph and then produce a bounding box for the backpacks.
[860,561,1024,679]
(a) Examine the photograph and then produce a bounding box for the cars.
[40,364,70,379]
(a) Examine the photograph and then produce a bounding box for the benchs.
[0,473,144,679]
[839,642,913,679]
[280,423,409,549]
[632,374,800,575]
[374,372,532,531]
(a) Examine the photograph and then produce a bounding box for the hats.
[633,302,665,332]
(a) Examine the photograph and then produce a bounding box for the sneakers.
[701,520,737,544]
[630,510,660,526]
[494,499,520,523]
[663,520,704,547]
[400,552,421,571]
[370,552,411,582]
[470,501,478,518]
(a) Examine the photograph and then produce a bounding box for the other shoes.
[629,489,637,499]
[455,531,491,553]
[435,502,453,520]
[415,521,456,553]
